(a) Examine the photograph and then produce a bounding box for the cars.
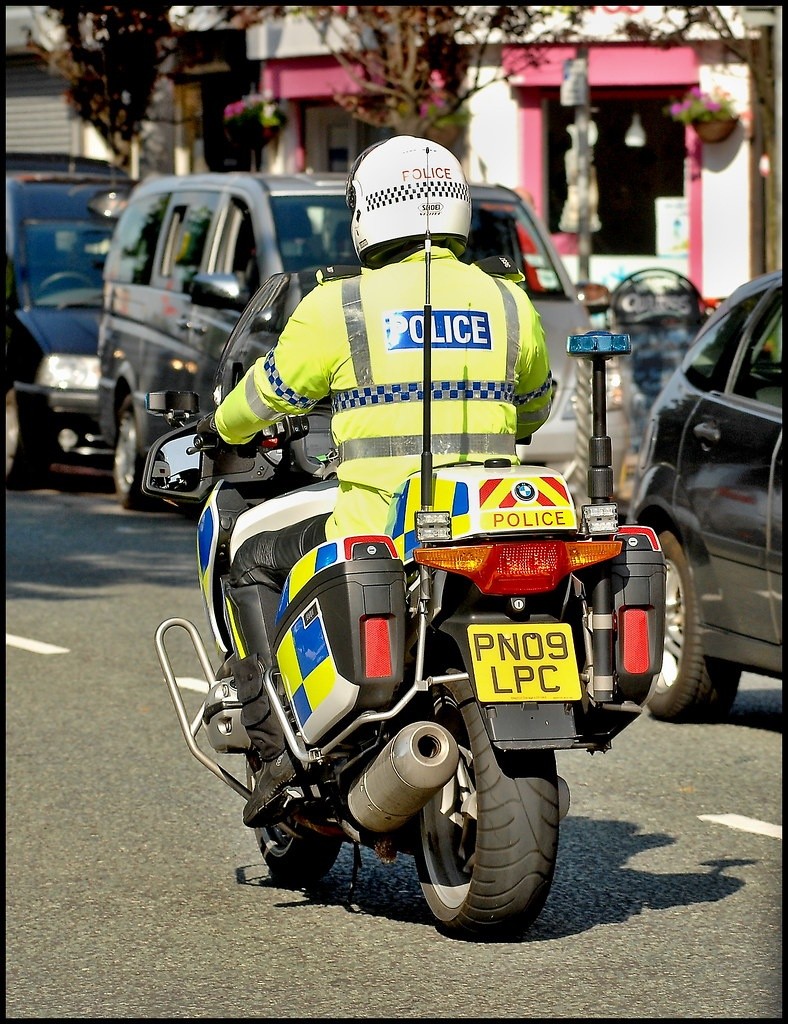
[603,272,787,721]
[568,266,718,524]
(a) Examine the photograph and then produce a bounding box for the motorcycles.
[136,265,672,944]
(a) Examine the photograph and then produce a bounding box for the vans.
[1,163,137,495]
[99,170,636,521]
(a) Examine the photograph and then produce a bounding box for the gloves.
[194,411,238,461]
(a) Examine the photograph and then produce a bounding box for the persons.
[192,132,560,828]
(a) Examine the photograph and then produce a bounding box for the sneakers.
[243,749,303,828]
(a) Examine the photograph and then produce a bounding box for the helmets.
[348,136,470,266]
